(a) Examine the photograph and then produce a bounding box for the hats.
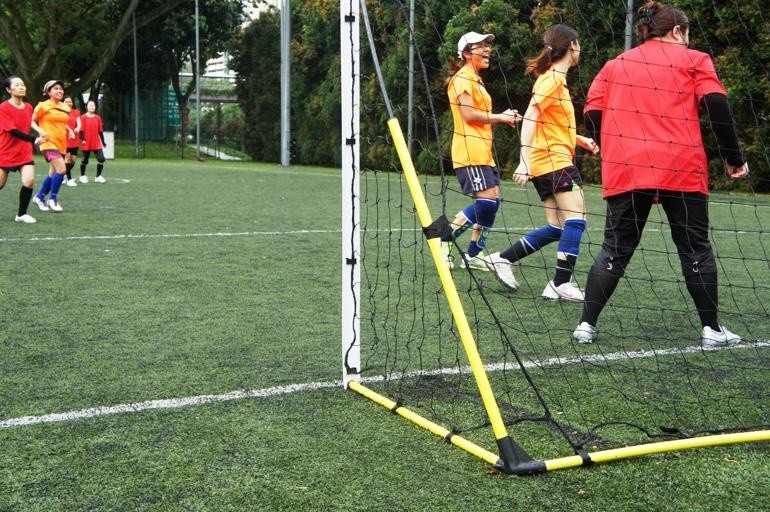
[43,80,64,96]
[458,31,495,61]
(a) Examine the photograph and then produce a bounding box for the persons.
[79,100,106,183]
[63,96,82,187]
[438,30,521,271]
[485,23,599,303]
[572,1,749,349]
[0,75,44,223]
[31,79,71,211]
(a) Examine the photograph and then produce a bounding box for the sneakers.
[484,252,520,290]
[79,175,88,183]
[62,175,77,187]
[47,199,63,211]
[442,241,455,269]
[573,321,597,344]
[542,276,585,302]
[15,214,37,224]
[461,251,489,271]
[32,196,49,211]
[701,325,740,347]
[95,175,106,183]
